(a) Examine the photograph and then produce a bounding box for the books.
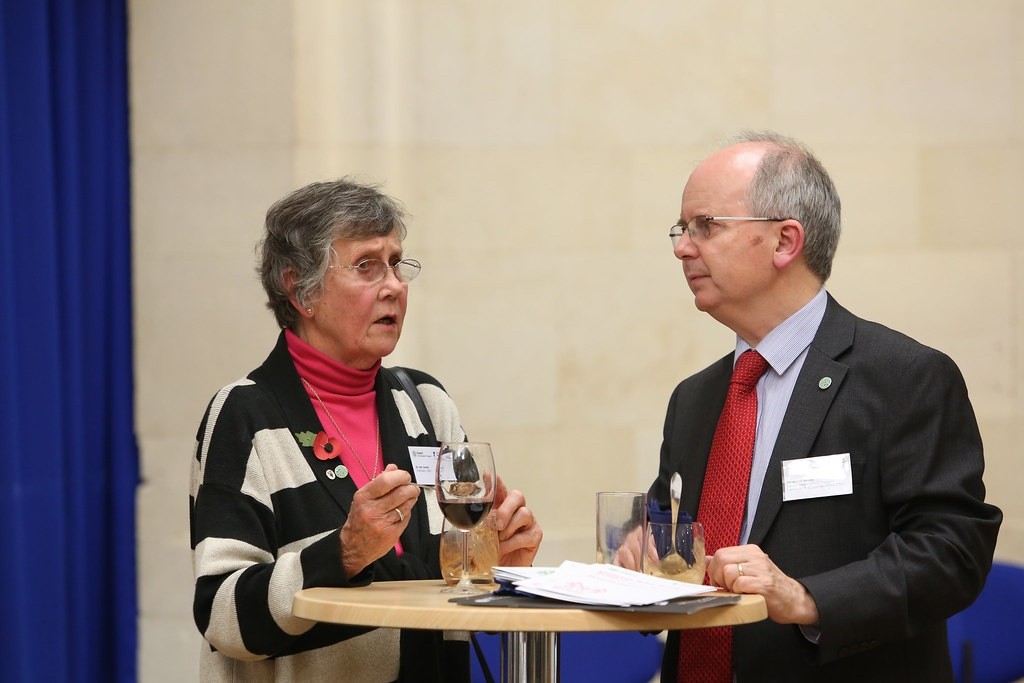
[451,592,742,615]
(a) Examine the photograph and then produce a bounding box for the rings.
[395,508,404,520]
[738,563,744,576]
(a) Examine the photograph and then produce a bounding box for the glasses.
[669,215,792,249]
[328,259,421,285]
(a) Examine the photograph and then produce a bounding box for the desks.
[291,579,770,682]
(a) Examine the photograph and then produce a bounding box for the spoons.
[371,475,481,498]
[657,472,687,576]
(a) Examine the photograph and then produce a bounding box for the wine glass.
[435,442,497,596]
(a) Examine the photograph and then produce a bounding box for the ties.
[679,350,770,683]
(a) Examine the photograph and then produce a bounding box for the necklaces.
[302,378,380,480]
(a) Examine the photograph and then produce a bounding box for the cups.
[439,506,499,585]
[596,492,647,573]
[643,522,706,587]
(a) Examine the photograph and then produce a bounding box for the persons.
[188,182,543,683]
[614,131,1003,683]
